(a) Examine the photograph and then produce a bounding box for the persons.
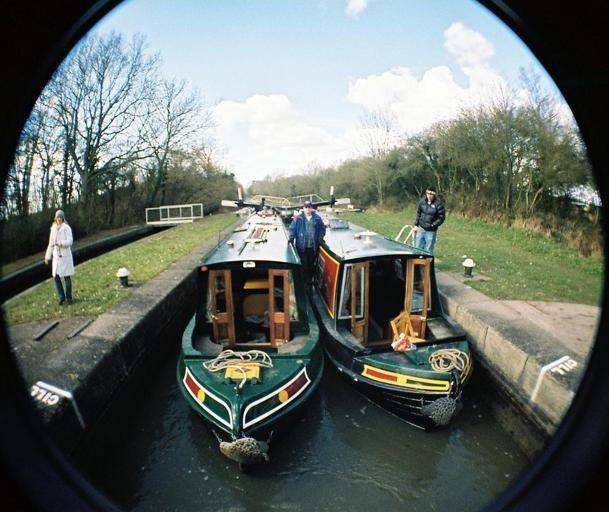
[415,184,446,256]
[286,200,327,297]
[43,208,76,308]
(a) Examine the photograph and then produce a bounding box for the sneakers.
[59,299,73,304]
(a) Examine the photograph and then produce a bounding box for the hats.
[302,201,312,208]
[425,185,438,195]
[55,209,65,218]
